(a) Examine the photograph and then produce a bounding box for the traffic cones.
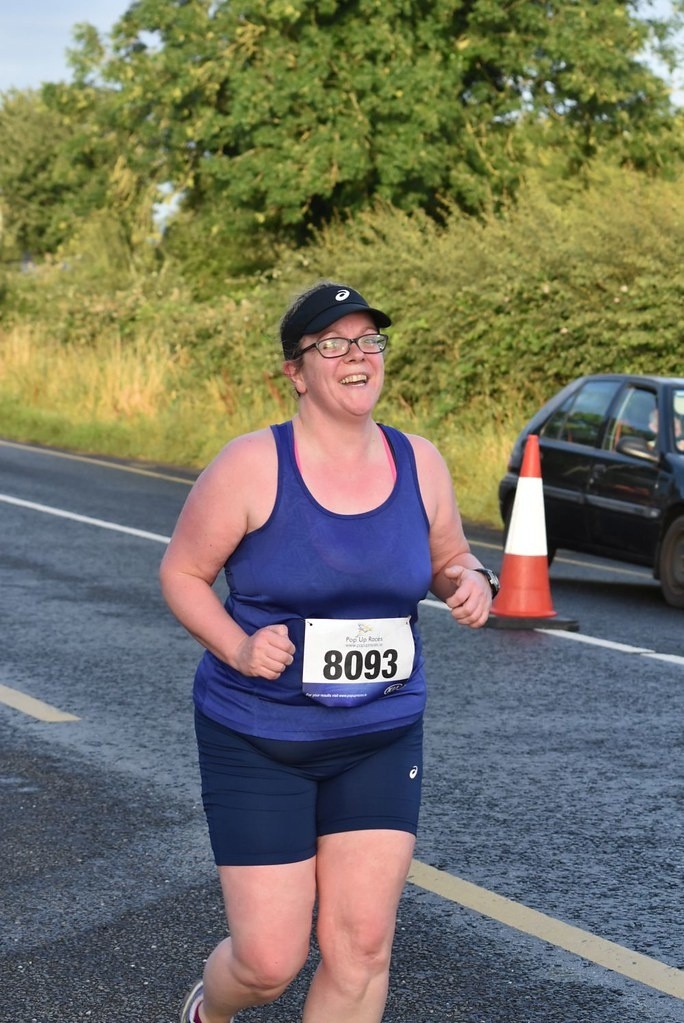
[486,434,584,633]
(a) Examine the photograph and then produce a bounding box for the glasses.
[292,334,389,360]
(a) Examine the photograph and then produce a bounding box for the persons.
[160,284,492,1023]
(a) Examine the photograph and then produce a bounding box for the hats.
[282,286,392,359]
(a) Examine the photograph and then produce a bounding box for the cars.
[498,373,684,612]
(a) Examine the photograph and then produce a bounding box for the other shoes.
[180,979,234,1023]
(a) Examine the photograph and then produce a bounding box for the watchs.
[475,568,500,599]
